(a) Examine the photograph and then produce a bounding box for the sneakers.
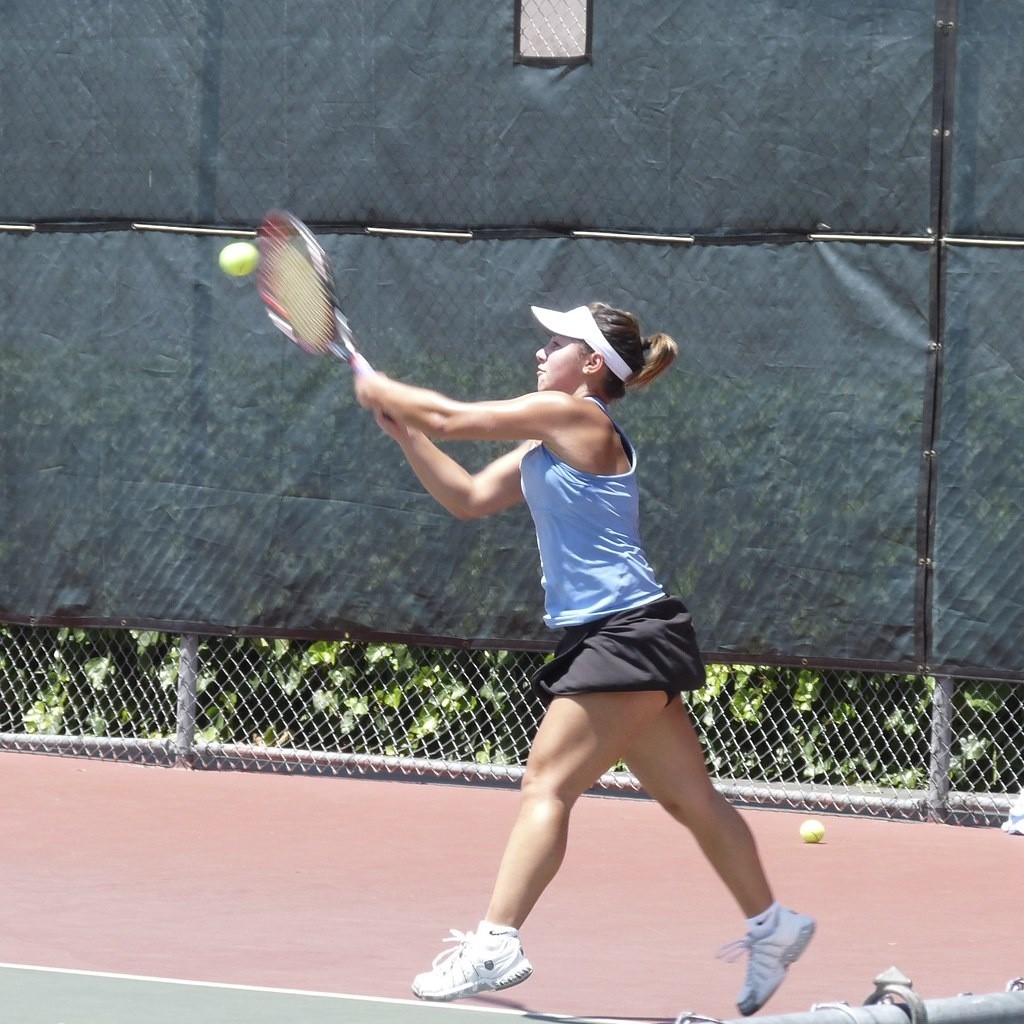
[411,929,534,1002]
[715,905,816,1017]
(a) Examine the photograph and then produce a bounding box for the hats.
[531,305,633,384]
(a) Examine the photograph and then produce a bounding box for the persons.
[355,302,814,1017]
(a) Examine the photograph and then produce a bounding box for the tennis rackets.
[251,205,400,426]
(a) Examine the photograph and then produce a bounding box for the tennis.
[216,240,262,278]
[798,819,828,843]
